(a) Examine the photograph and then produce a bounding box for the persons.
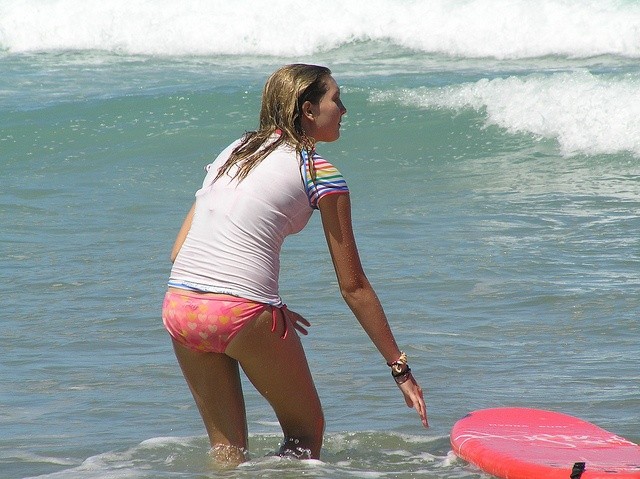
[161,63,430,467]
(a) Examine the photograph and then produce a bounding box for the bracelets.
[386,351,412,384]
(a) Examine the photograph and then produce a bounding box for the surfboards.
[450,407,640,479]
